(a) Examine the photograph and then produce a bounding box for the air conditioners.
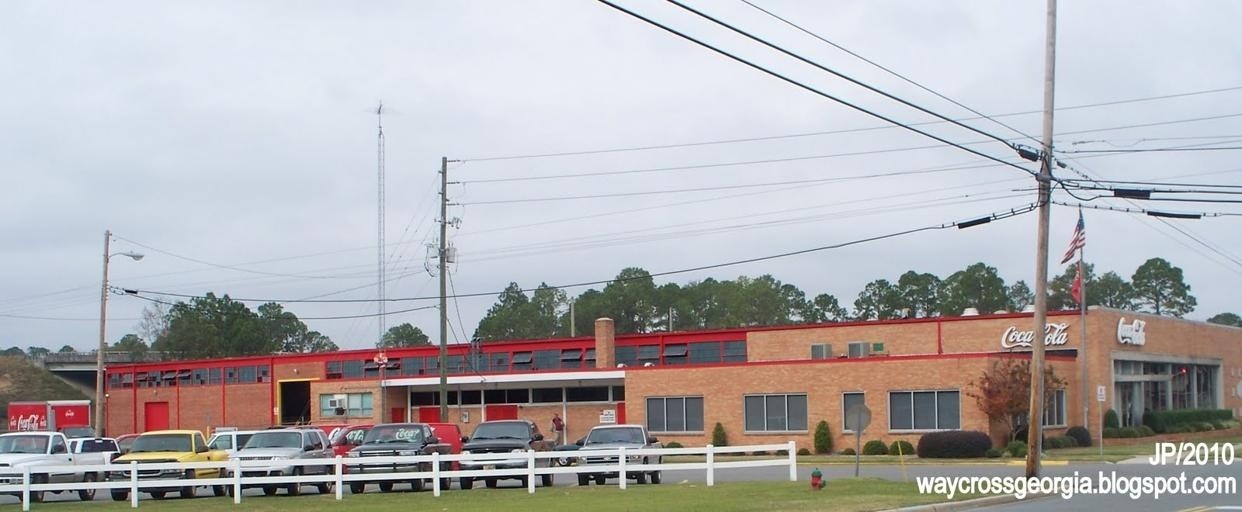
[811,344,832,360]
[848,341,870,358]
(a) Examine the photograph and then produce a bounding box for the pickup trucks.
[576,425,662,486]
[0,432,105,503]
[458,420,556,489]
[345,423,452,491]
[108,429,227,501]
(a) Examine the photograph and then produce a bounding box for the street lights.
[94,229,144,437]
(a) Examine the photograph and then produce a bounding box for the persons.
[550,413,563,446]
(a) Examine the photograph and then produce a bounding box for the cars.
[58,438,122,481]
[553,435,586,466]
[116,434,140,453]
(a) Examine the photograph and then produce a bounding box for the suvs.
[227,430,335,495]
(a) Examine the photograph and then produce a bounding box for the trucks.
[8,401,96,437]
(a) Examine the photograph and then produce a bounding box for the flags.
[1061,209,1086,264]
[1071,261,1081,304]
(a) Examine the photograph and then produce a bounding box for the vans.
[269,424,349,443]
[332,423,463,473]
[208,429,255,474]
